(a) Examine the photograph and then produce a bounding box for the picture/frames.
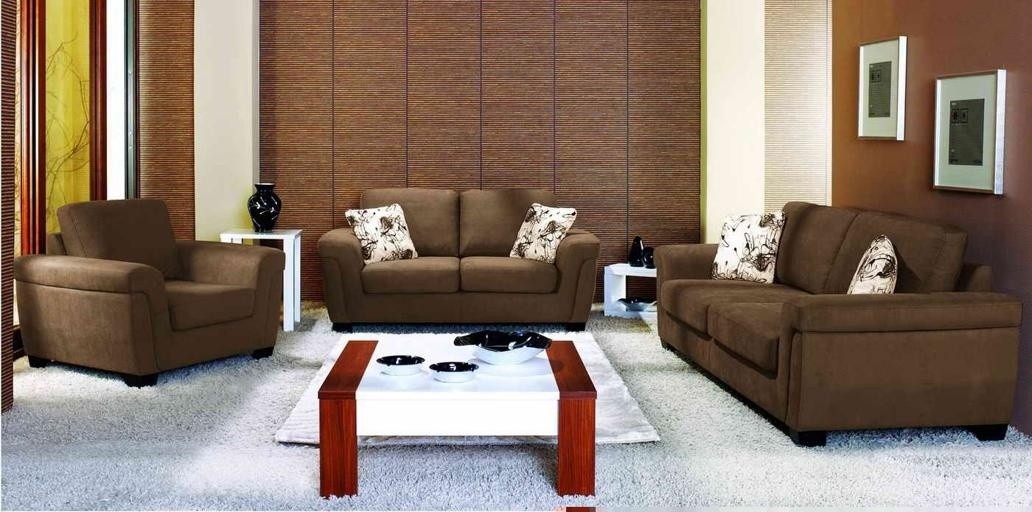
[855,35,907,142]
[931,68,1006,196]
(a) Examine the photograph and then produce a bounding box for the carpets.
[274,329,662,446]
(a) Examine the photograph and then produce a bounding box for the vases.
[630,236,643,267]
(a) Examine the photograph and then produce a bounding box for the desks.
[220,227,305,333]
[604,263,659,319]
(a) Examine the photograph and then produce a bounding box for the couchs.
[314,186,603,333]
[651,201,1024,448]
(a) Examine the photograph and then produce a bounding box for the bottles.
[628,236,645,266]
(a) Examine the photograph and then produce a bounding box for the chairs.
[12,197,287,390]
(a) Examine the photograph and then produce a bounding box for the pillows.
[846,232,899,296]
[343,203,419,265]
[709,211,786,284]
[509,201,578,264]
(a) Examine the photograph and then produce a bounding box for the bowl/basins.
[429,362,480,382]
[376,354,427,375]
[453,328,552,368]
[617,296,658,311]
